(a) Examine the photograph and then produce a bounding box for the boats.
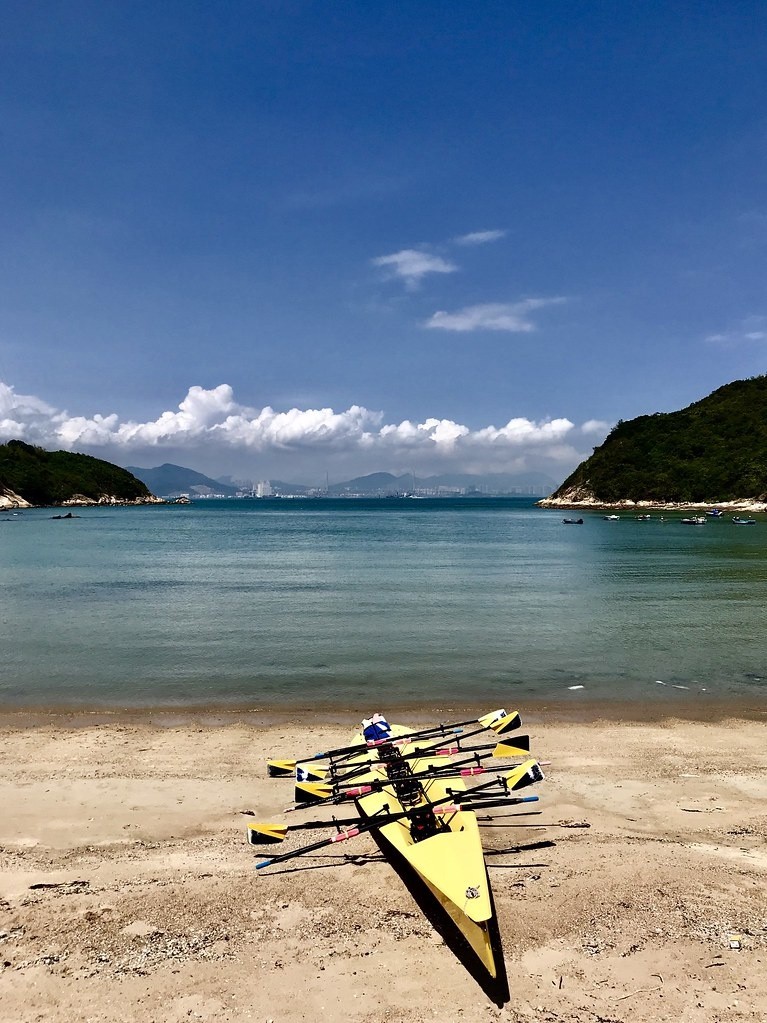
[705,508,724,517]
[635,513,651,522]
[679,514,708,525]
[345,711,498,980]
[731,516,757,525]
[561,518,583,524]
[605,514,621,521]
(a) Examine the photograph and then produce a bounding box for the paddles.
[246,705,545,871]
